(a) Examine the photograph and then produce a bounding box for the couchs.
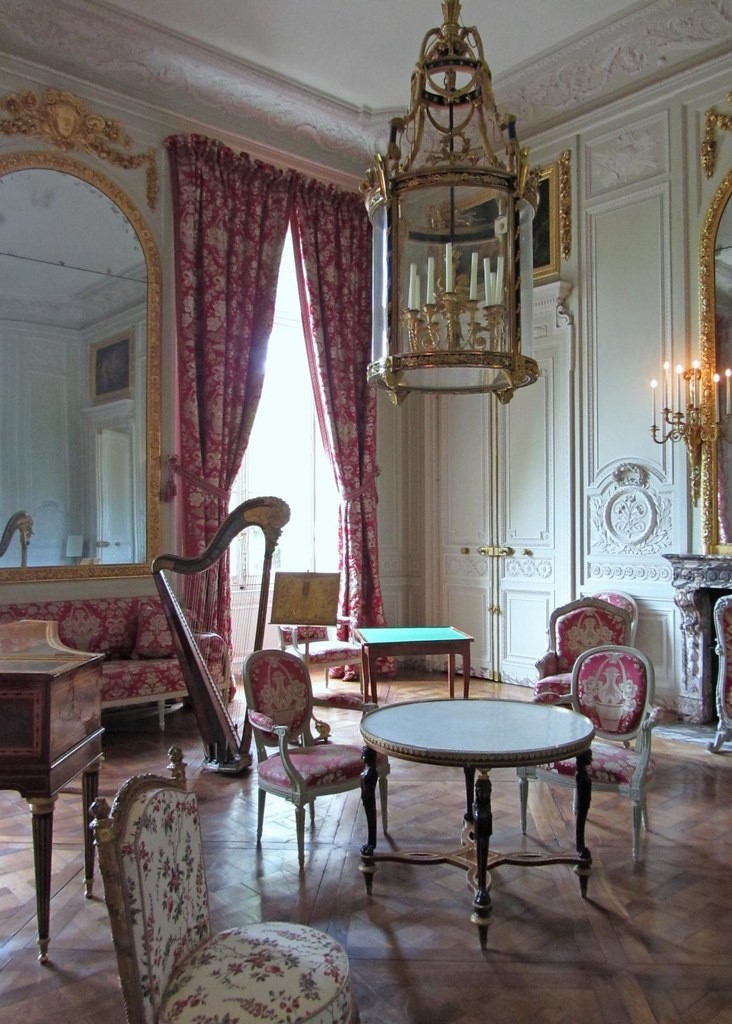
[0,594,231,732]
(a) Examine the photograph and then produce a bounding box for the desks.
[359,698,595,951]
[0,620,105,962]
[661,554,732,724]
[353,626,474,703]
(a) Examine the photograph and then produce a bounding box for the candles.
[651,368,731,425]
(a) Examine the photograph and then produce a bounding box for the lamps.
[358,0,546,409]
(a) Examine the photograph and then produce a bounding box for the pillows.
[131,601,196,659]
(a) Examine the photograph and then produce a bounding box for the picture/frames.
[89,325,135,406]
[426,150,572,311]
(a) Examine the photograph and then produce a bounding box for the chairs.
[278,625,363,694]
[243,650,390,869]
[515,645,659,863]
[534,597,632,705]
[706,593,732,753]
[590,590,639,647]
[88,745,361,1024]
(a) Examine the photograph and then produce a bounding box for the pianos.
[0,620,107,965]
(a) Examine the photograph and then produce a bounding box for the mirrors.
[0,85,163,585]
